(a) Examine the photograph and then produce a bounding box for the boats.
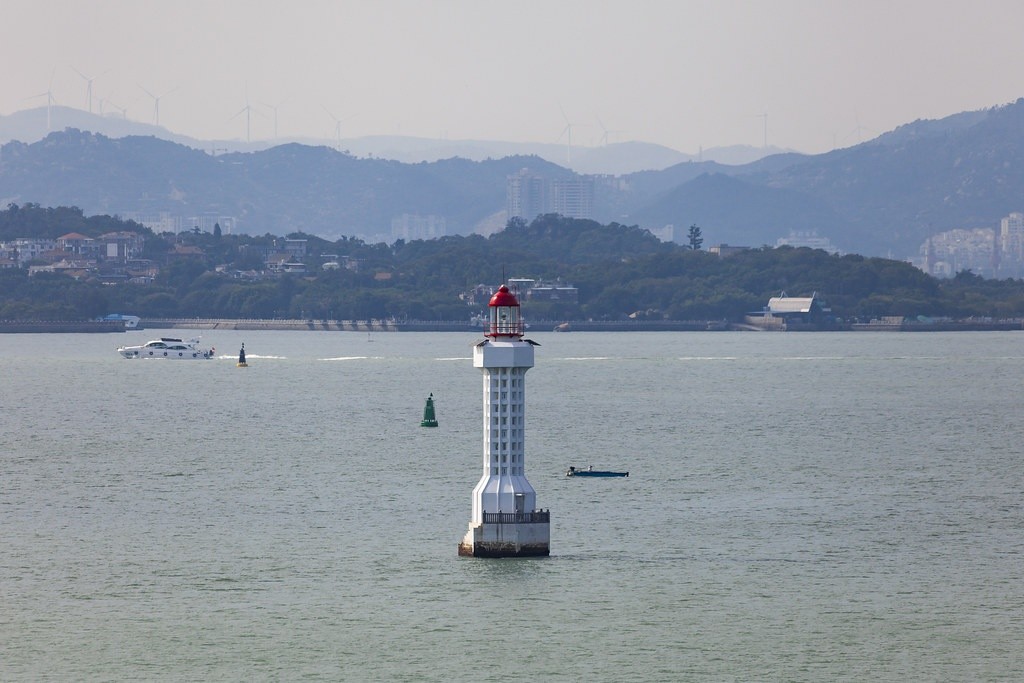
[554,322,572,332]
[566,465,629,477]
[118,338,216,359]
